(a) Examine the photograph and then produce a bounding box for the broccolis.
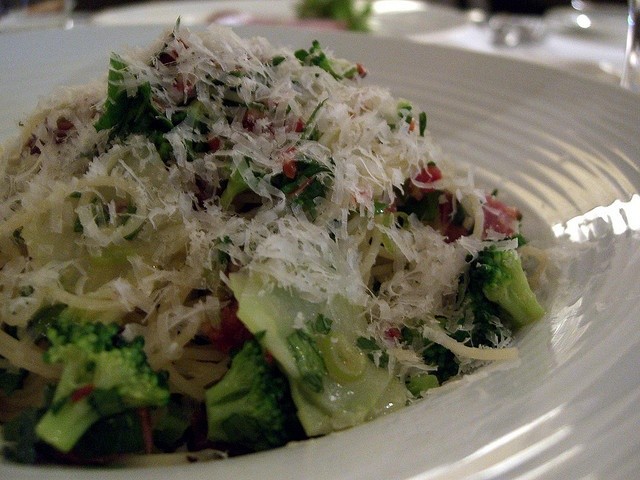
[471,246,545,329]
[204,346,285,440]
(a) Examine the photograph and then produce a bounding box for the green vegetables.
[33,311,168,454]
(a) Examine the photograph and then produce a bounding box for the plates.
[0,23,639,480]
